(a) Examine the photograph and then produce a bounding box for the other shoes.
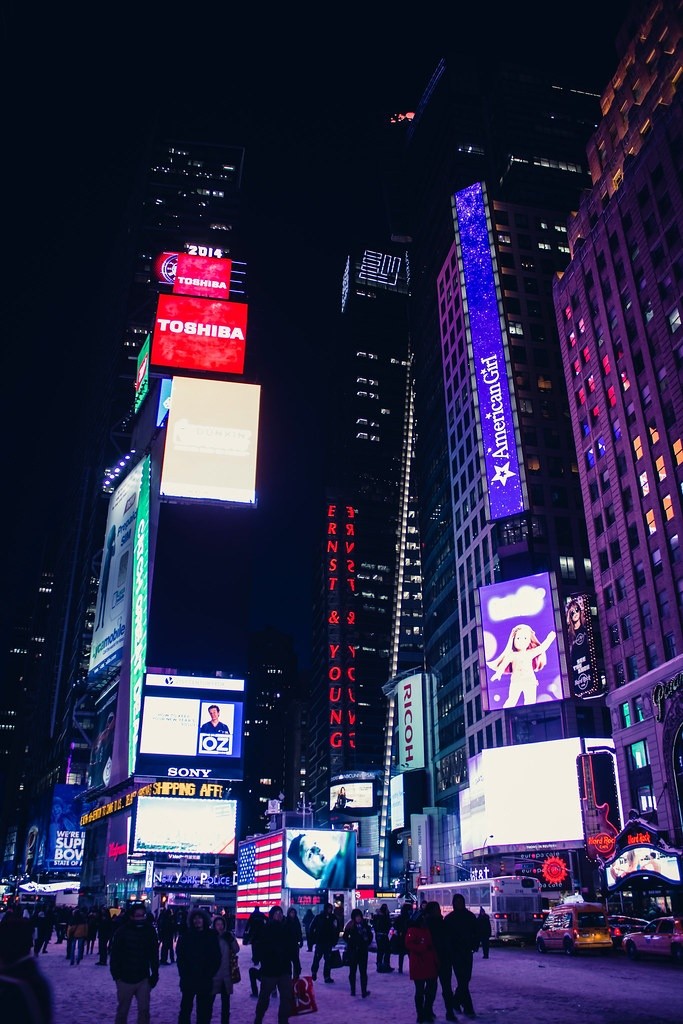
[325,978,333,983]
[35,947,107,966]
[464,1008,476,1018]
[483,954,489,959]
[312,974,316,980]
[250,994,259,998]
[453,997,461,1014]
[376,962,395,973]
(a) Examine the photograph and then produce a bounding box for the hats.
[351,909,363,919]
[269,906,283,915]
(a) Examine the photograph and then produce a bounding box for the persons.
[0,899,491,1024]
[289,833,327,878]
[642,852,661,871]
[610,850,640,882]
[52,797,80,830]
[201,705,229,733]
[609,907,672,920]
[486,624,557,708]
[337,787,347,808]
[567,601,586,652]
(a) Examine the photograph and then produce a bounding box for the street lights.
[478,834,494,880]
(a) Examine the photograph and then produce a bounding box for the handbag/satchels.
[342,951,350,966]
[331,950,342,968]
[230,955,240,983]
[291,977,317,1016]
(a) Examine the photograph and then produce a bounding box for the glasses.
[134,914,146,917]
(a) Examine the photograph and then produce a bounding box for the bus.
[416,874,544,947]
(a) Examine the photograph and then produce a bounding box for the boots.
[349,974,356,996]
[415,996,424,1022]
[424,993,434,1022]
[443,992,457,1021]
[360,976,370,998]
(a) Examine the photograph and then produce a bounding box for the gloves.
[222,932,234,943]
[299,942,303,948]
[149,972,159,987]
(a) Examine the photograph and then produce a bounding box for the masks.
[133,920,144,925]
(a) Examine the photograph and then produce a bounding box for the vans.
[535,901,614,956]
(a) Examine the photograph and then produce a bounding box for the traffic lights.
[435,866,441,876]
[499,861,505,871]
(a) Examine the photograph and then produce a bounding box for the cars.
[620,915,683,964]
[602,914,658,950]
[367,912,400,953]
